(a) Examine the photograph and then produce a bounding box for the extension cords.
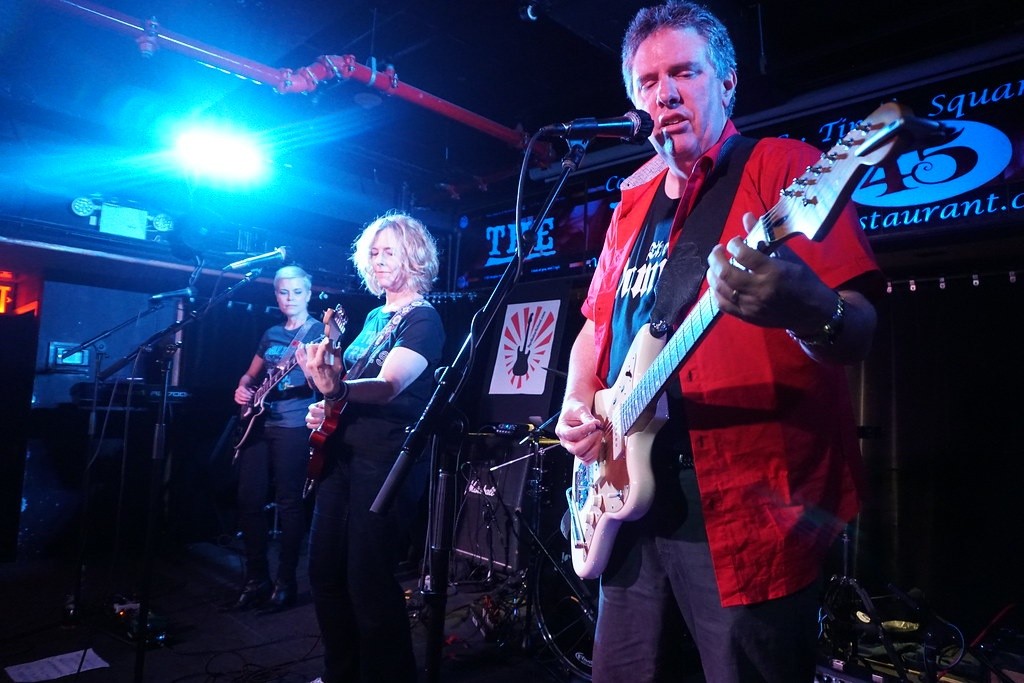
[472,615,507,640]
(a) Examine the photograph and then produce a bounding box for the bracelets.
[324,381,349,403]
[786,293,846,347]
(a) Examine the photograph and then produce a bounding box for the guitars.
[301,309,348,498]
[562,101,966,582]
[232,303,348,450]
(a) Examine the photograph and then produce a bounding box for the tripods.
[809,341,907,683]
[0,266,306,683]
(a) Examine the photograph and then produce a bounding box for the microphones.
[222,245,296,272]
[539,109,654,139]
[151,287,199,299]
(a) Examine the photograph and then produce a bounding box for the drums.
[531,525,600,683]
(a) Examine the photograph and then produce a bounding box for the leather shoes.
[217,575,268,610]
[258,577,297,614]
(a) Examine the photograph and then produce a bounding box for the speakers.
[452,433,575,575]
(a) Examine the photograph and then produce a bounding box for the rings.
[731,289,740,305]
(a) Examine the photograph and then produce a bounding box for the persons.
[214,265,337,613]
[305,207,447,683]
[554,0,880,683]
